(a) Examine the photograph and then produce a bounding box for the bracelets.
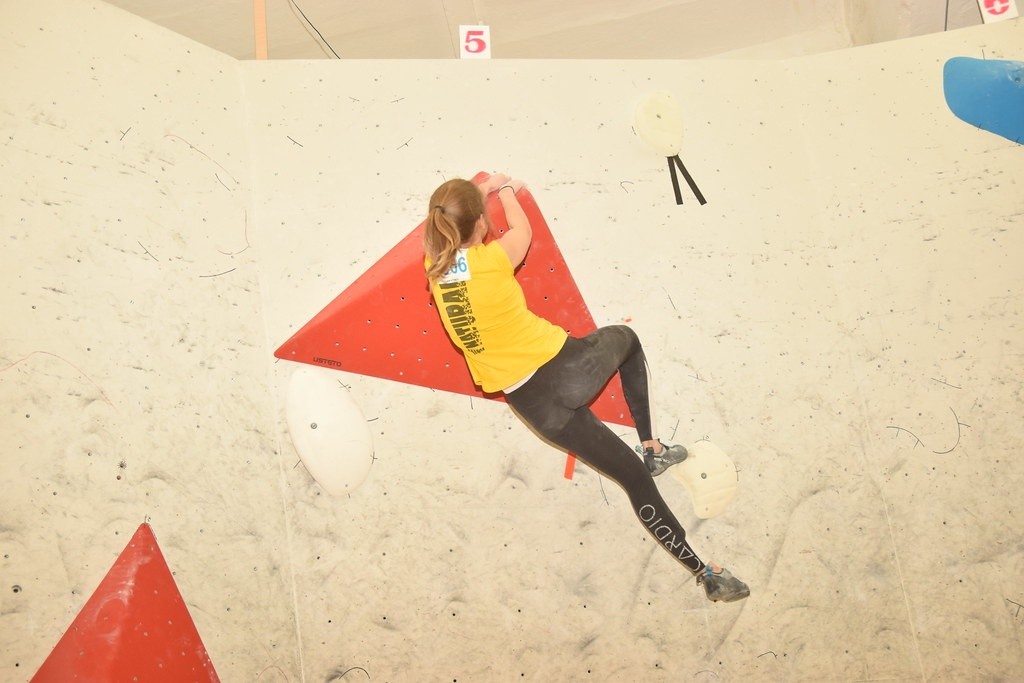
[498,185,514,193]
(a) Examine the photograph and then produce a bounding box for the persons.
[425,172,751,602]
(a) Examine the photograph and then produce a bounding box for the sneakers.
[696,566,750,603]
[643,438,688,477]
